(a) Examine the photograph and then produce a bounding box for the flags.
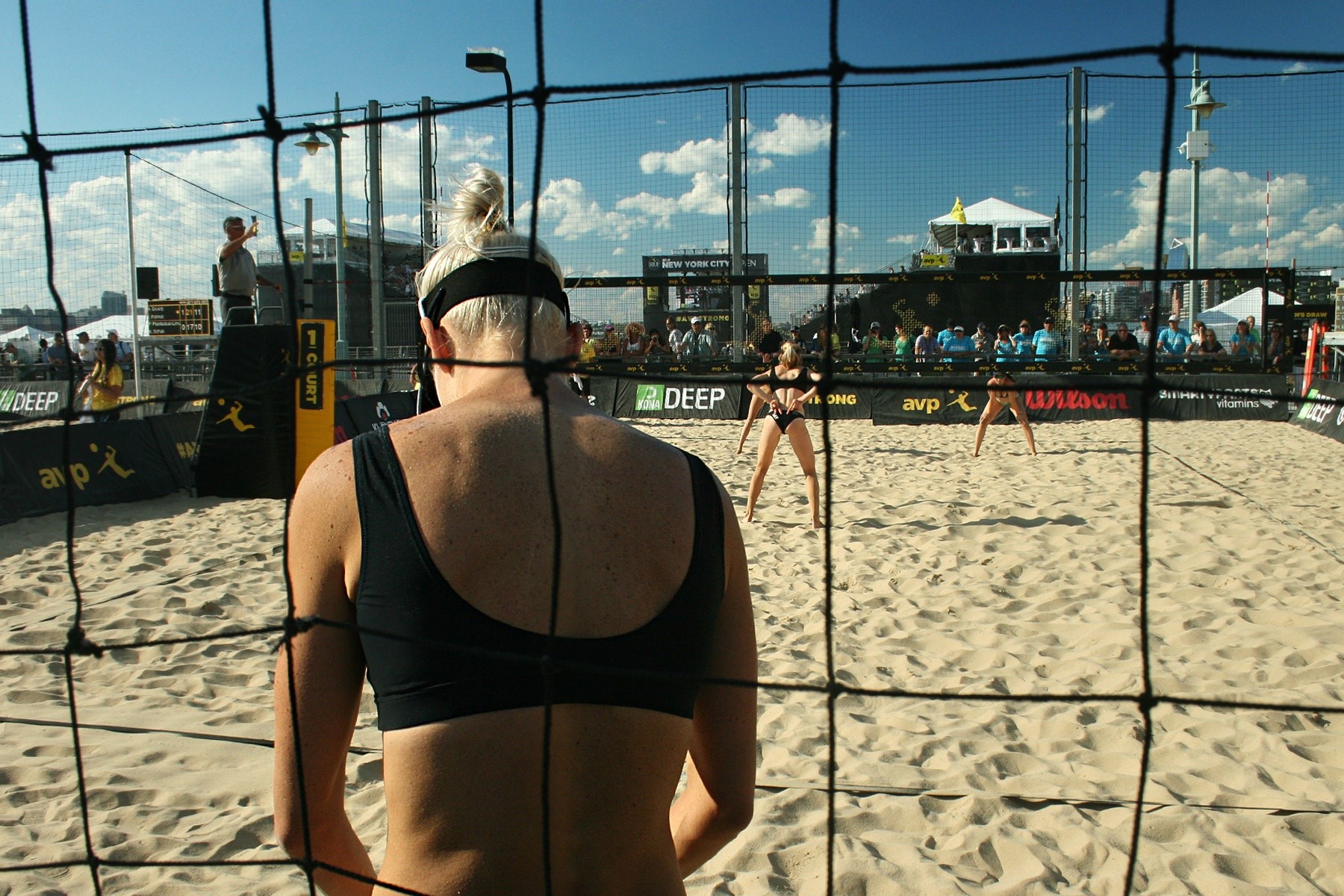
[950,196,966,224]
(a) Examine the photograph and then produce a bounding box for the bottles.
[677,350,683,360]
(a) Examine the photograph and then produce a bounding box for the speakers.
[136,267,159,300]
[211,264,221,297]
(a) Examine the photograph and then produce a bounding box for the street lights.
[465,47,515,234]
[1181,52,1228,334]
[292,90,349,378]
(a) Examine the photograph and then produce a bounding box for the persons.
[568,234,1309,528]
[215,216,281,325]
[0,328,134,422]
[272,161,757,896]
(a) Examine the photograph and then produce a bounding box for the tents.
[1178,288,1302,354]
[0,315,223,360]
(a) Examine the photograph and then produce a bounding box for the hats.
[1098,323,1108,329]
[413,257,572,411]
[106,329,120,338]
[954,326,964,332]
[625,322,645,336]
[1238,319,1248,325]
[1,343,12,351]
[691,316,701,324]
[604,324,614,331]
[1168,314,1180,322]
[870,322,880,330]
[76,332,89,339]
[791,326,800,333]
[1044,317,1053,324]
[1141,315,1149,321]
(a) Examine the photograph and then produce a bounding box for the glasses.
[1205,334,1213,337]
[995,375,1005,379]
[231,226,246,230]
[1118,329,1127,332]
[94,346,104,352]
[710,328,715,330]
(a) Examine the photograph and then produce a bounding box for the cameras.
[653,337,657,341]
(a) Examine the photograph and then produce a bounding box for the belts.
[221,292,253,301]
